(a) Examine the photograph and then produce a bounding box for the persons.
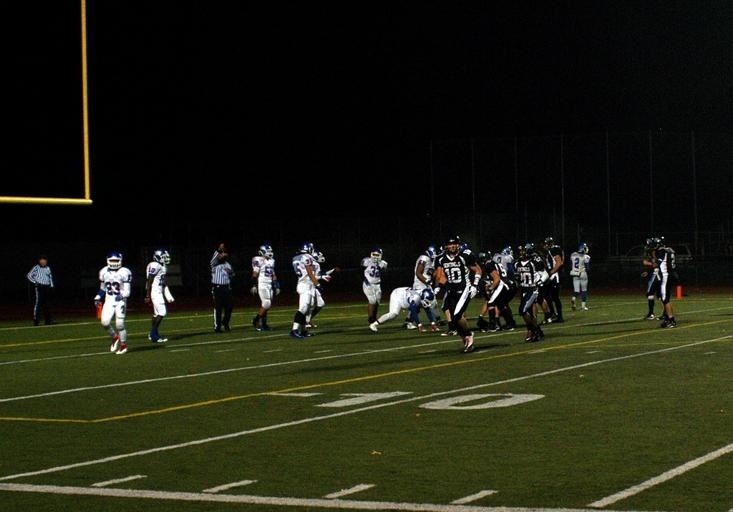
[26,256,56,326]
[403,235,565,354]
[640,236,677,322]
[209,241,234,333]
[250,243,281,333]
[359,246,389,332]
[290,242,321,338]
[304,251,336,328]
[144,248,176,343]
[569,242,593,311]
[368,285,440,334]
[92,250,134,356]
[654,235,680,329]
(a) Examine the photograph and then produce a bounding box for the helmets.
[518,237,553,259]
[107,251,122,270]
[420,289,434,309]
[368,247,382,263]
[152,248,170,265]
[426,234,468,259]
[258,245,273,258]
[478,236,514,263]
[300,242,325,263]
[577,243,589,254]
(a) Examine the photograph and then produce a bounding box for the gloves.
[94,300,100,308]
[249,287,257,296]
[143,296,150,305]
[430,322,440,333]
[416,320,427,334]
[275,288,280,296]
[115,295,122,301]
[467,286,478,300]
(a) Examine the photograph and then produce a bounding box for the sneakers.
[109,335,119,352]
[147,332,167,343]
[581,307,588,310]
[368,323,377,333]
[440,328,458,336]
[289,322,317,338]
[570,299,577,311]
[525,326,543,342]
[542,313,563,324]
[253,317,272,332]
[476,319,517,333]
[642,313,677,329]
[463,331,474,353]
[115,344,127,355]
[403,321,417,330]
